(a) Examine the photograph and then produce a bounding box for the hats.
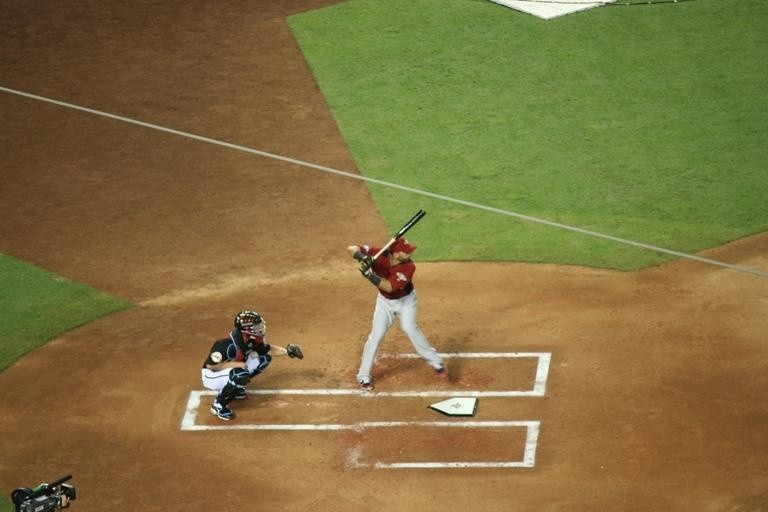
[387,238,418,256]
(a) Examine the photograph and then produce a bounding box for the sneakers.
[432,363,447,379]
[233,391,250,399]
[208,402,237,421]
[360,379,376,391]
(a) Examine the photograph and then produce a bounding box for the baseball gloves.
[287,344,303,359]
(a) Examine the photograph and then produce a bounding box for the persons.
[200,310,304,421]
[347,235,450,392]
[10,481,76,512]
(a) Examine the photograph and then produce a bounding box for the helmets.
[234,310,262,337]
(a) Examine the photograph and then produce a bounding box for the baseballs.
[211,352,223,363]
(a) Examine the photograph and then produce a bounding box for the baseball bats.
[359,209,425,272]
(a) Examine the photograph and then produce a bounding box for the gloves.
[358,264,381,287]
[285,343,304,362]
[352,248,375,266]
